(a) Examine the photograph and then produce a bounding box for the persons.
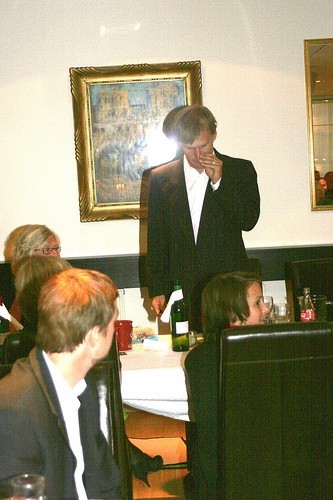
[146,106,260,332]
[0,226,163,487]
[315,171,333,205]
[184,272,270,487]
[0,270,133,500]
[3,255,75,331]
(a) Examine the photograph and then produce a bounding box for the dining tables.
[118,334,191,470]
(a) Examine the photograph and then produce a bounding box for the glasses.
[33,246,61,254]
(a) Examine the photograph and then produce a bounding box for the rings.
[213,161,215,165]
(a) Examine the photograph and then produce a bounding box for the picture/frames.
[68,61,202,220]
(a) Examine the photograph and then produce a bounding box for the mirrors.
[304,38,333,212]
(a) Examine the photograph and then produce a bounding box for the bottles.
[300,288,315,322]
[170,282,189,351]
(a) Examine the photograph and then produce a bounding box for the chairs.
[284,257,332,321]
[0,331,134,500]
[218,319,333,500]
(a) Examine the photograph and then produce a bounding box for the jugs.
[298,295,333,322]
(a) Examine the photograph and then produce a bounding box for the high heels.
[132,454,164,488]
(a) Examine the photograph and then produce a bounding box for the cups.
[114,320,133,350]
[263,297,293,324]
[10,473,45,500]
